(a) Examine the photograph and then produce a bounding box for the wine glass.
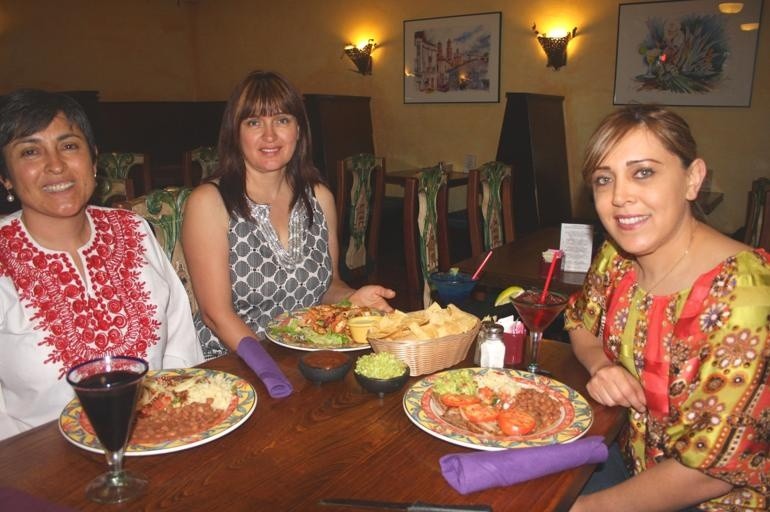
[65,353,152,507]
[509,289,571,378]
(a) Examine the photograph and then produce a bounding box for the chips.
[366,300,479,342]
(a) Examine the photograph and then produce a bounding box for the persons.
[180,70,396,354]
[562,104,768,512]
[1,88,205,444]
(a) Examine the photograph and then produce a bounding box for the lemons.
[494,286,525,307]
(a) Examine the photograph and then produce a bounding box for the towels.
[438,434,609,495]
[236,336,294,398]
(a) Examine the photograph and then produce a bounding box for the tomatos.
[465,404,502,422]
[442,394,482,407]
[500,412,537,436]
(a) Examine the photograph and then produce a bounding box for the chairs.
[93,149,152,207]
[744,178,770,254]
[338,155,385,272]
[467,161,516,258]
[404,173,450,311]
[182,145,221,188]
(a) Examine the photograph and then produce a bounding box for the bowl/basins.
[346,316,381,346]
[298,350,354,384]
[353,363,410,396]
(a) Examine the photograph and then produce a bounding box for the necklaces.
[634,229,695,310]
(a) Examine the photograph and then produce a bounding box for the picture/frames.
[612,1,763,107]
[402,11,501,104]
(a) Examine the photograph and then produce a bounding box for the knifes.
[318,495,493,511]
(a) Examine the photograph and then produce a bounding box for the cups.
[499,330,527,366]
[430,268,479,306]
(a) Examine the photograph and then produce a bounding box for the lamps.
[342,39,372,75]
[532,24,579,69]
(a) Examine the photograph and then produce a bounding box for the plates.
[402,366,596,451]
[264,306,374,352]
[57,367,259,458]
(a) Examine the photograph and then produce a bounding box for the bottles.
[473,320,506,369]
